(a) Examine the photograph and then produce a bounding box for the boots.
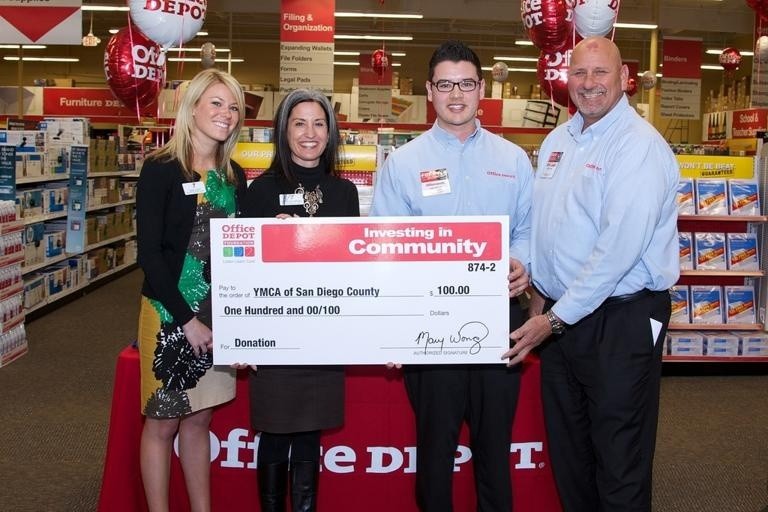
[290,459,319,511]
[257,458,288,511]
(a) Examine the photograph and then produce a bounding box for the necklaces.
[289,163,324,216]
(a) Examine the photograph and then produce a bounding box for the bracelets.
[545,309,566,334]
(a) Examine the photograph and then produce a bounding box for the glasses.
[430,78,480,92]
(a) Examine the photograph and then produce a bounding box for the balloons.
[536,34,583,108]
[200,42,216,67]
[755,36,768,62]
[104,24,166,113]
[520,0,575,54]
[573,0,619,39]
[626,78,638,97]
[641,71,657,89]
[718,47,743,70]
[127,0,208,47]
[370,48,389,76]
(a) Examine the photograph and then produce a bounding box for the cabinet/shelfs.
[658,155,768,365]
[0,112,142,367]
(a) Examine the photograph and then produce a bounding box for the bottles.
[0,200,30,366]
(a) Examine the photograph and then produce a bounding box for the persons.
[499,36,682,512]
[365,40,536,512]
[133,68,249,511]
[240,86,361,512]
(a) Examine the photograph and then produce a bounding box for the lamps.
[0,1,758,83]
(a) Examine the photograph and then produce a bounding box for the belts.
[599,291,651,307]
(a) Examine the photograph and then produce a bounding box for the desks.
[98,344,560,510]
